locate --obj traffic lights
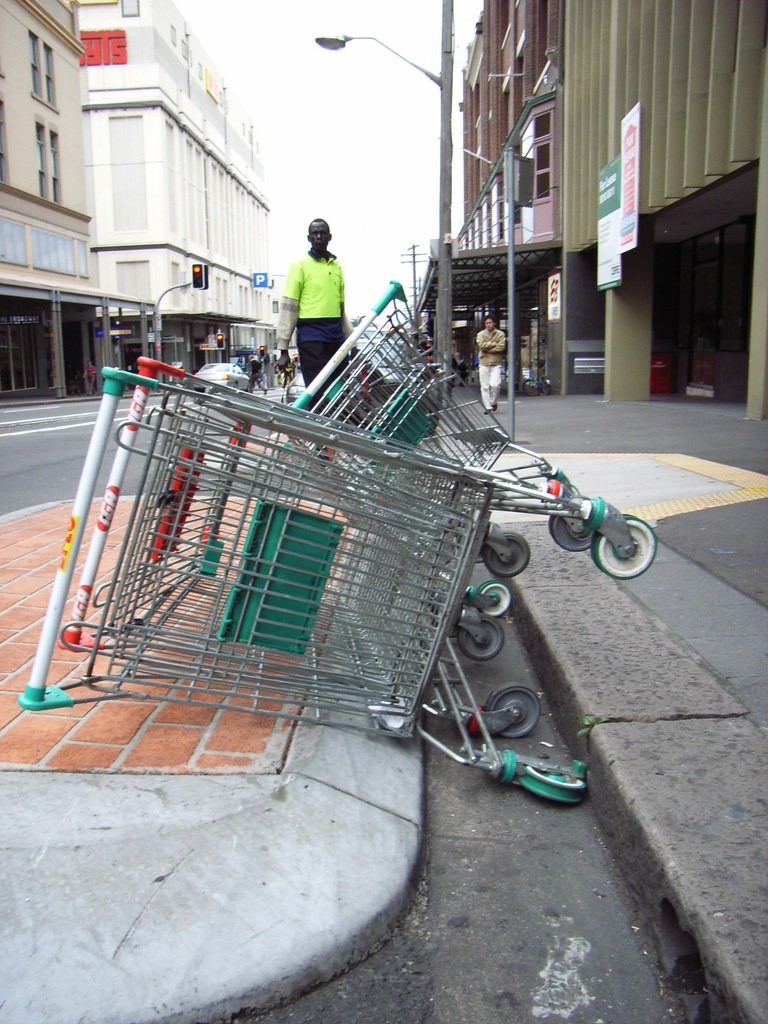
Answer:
[192,263,204,289]
[217,333,224,350]
[259,345,266,357]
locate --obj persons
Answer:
[476,315,505,414]
[276,218,360,468]
[4,363,9,389]
[248,354,267,395]
[68,375,81,395]
[82,361,96,395]
[451,353,467,388]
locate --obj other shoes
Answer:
[492,405,497,411]
[484,410,491,414]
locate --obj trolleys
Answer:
[15,283,665,810]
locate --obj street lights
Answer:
[314,34,454,396]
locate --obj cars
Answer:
[193,362,251,393]
